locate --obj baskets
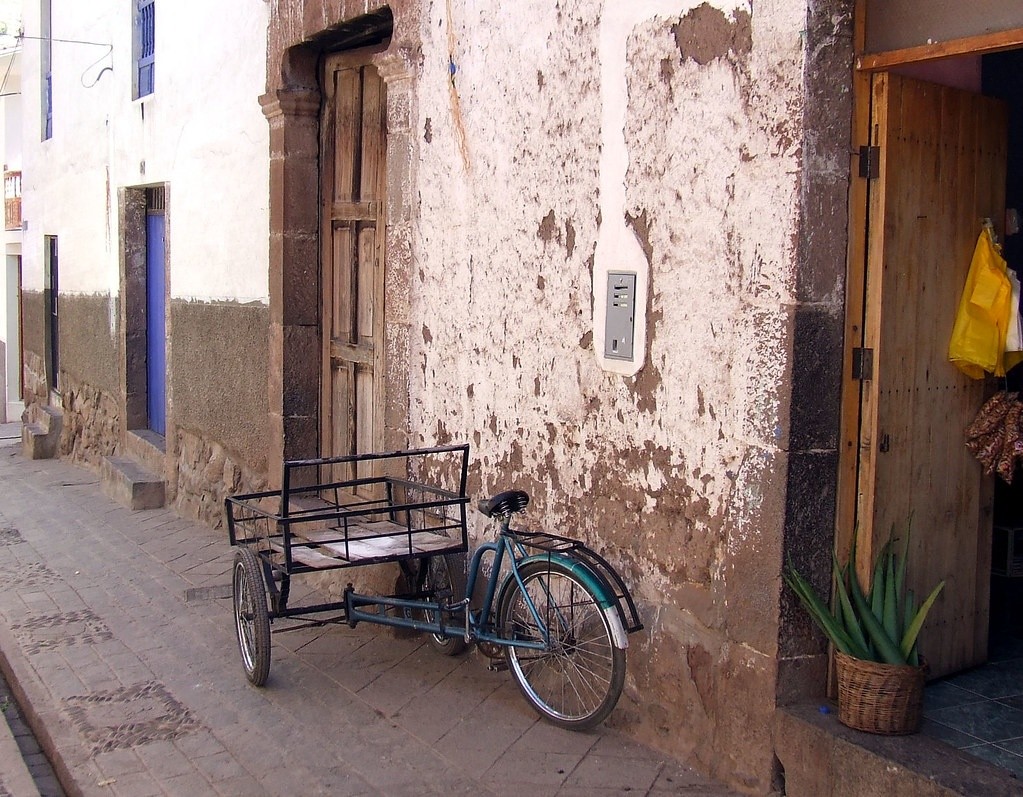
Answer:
[832,646,930,737]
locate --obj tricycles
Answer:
[222,443,644,733]
